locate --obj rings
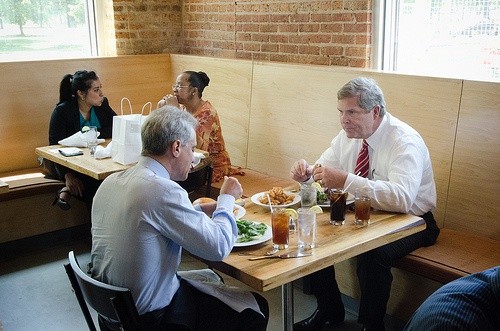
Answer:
[320,179,324,185]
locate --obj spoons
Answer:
[238,248,278,256]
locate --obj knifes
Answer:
[249,254,310,260]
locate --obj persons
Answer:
[290,77,440,331]
[40,71,120,213]
[86,104,269,331]
[157,70,231,184]
[404,267,500,331]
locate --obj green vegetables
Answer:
[316,191,328,205]
[235,219,266,243]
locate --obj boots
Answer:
[360,323,385,331]
[293,291,344,331]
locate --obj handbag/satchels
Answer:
[112,96,151,167]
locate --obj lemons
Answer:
[309,206,323,213]
[311,182,321,191]
[82,126,90,131]
[285,209,298,219]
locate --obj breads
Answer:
[193,198,214,205]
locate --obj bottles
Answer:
[86,126,97,156]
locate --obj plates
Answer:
[233,221,272,248]
[233,203,247,220]
[250,191,301,210]
[317,189,356,207]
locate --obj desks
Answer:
[35,138,209,179]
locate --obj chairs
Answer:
[63,250,142,331]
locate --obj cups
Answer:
[300,187,317,208]
[329,189,348,226]
[297,207,316,250]
[353,188,373,225]
[271,207,290,250]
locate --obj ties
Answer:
[354,139,368,178]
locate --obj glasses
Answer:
[172,84,193,90]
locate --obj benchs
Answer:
[0,54,500,320]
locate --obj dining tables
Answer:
[187,185,426,331]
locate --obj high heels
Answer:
[51,187,72,210]
